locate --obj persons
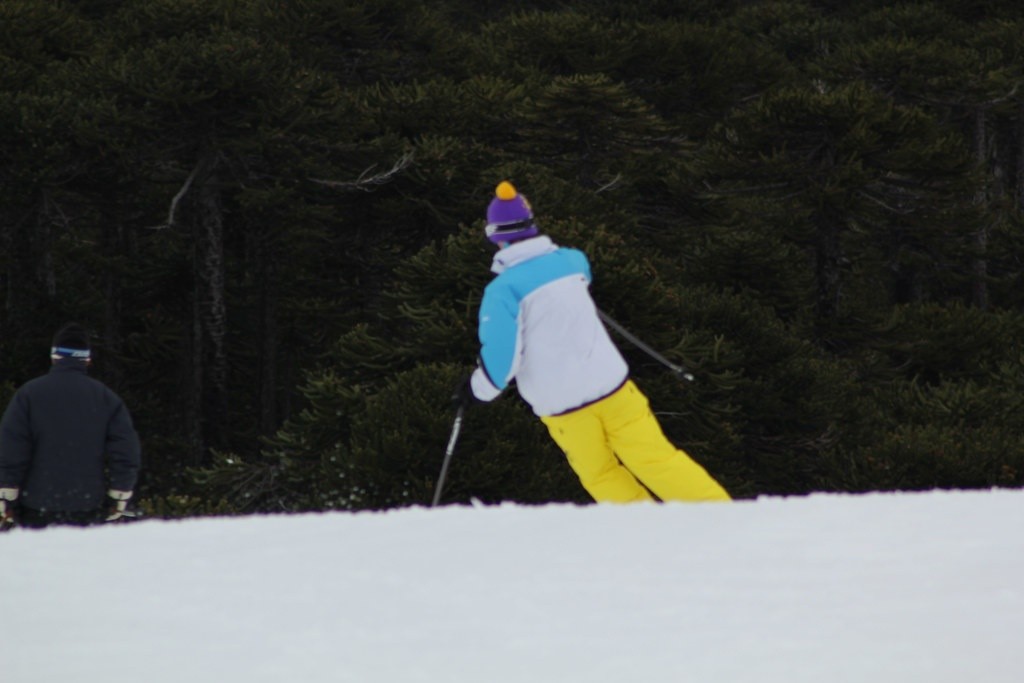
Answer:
[1,325,142,530]
[452,182,733,503]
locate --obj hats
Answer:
[484,180,537,243]
[49,324,91,361]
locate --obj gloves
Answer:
[0,499,14,523]
[103,500,128,521]
